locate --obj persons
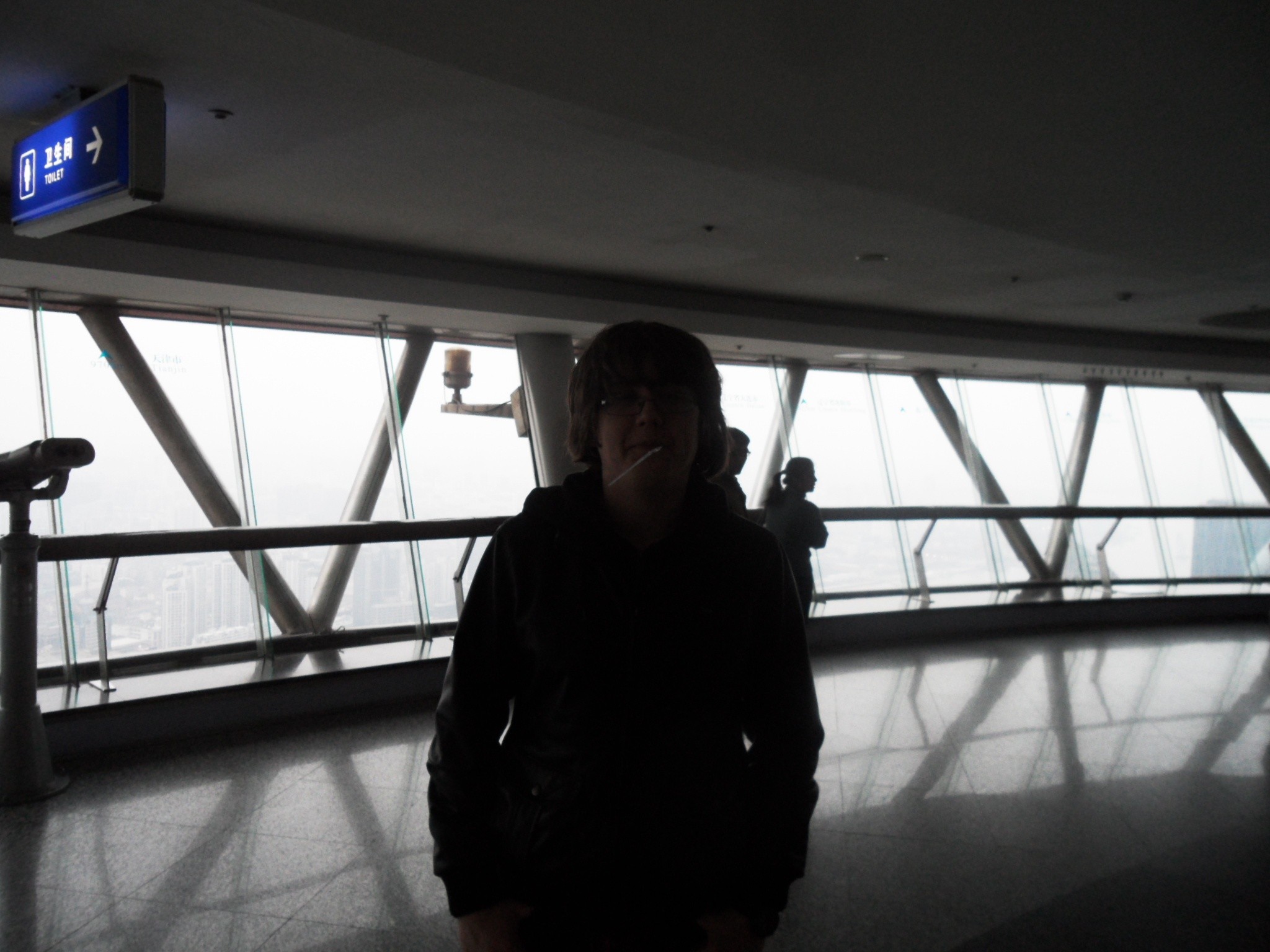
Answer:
[423,319,828,952]
[721,424,752,522]
[761,456,828,628]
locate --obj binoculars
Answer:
[0,437,97,807]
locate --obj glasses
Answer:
[597,390,701,417]
[731,448,751,458]
